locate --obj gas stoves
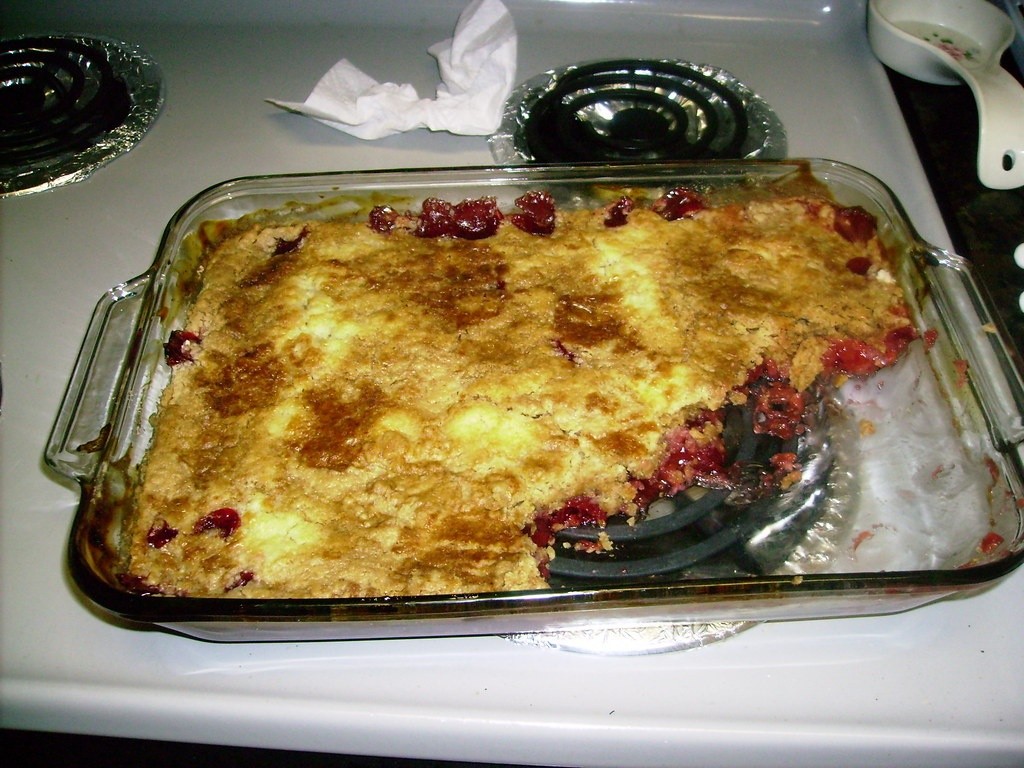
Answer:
[1,1,1022,767]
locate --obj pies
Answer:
[124,187,918,597]
